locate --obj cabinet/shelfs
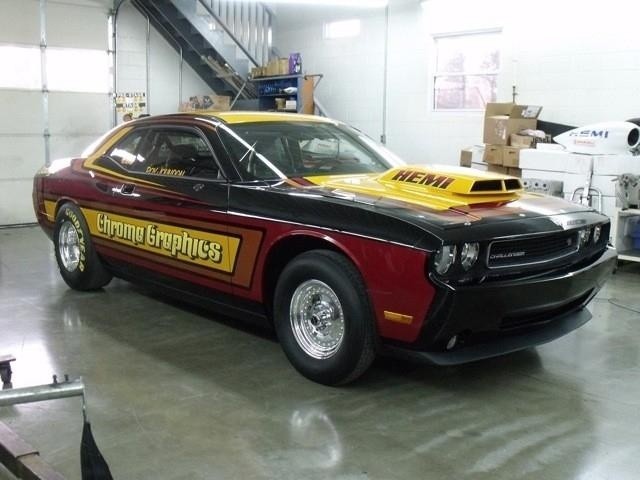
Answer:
[250,75,315,115]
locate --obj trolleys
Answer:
[568,184,639,276]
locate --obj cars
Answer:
[28,107,623,391]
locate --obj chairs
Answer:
[167,143,198,170]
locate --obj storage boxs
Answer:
[481,103,543,178]
[266,54,290,76]
[180,94,230,112]
